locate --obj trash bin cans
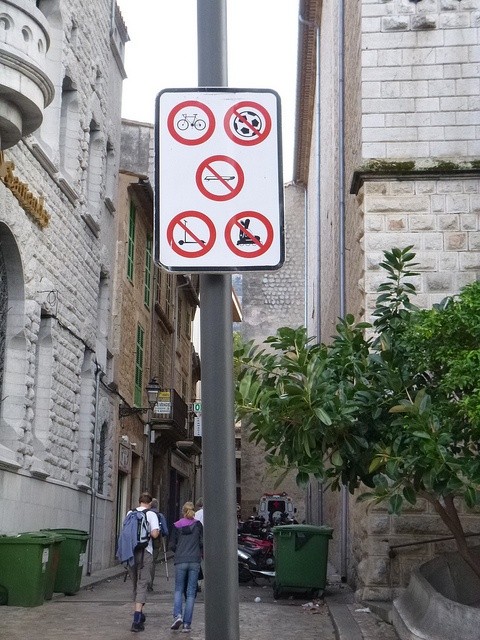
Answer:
[0,531,66,607]
[40,528,89,593]
[271,525,334,600]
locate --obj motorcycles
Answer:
[234,515,309,585]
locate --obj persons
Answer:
[195,498,203,526]
[114,493,161,632]
[146,498,168,592]
[169,501,203,632]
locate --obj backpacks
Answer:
[152,512,163,549]
[131,508,151,550]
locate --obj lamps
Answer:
[118,374,160,417]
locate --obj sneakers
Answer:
[170,614,183,629]
[181,623,192,632]
[130,622,144,632]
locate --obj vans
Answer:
[256,491,297,523]
